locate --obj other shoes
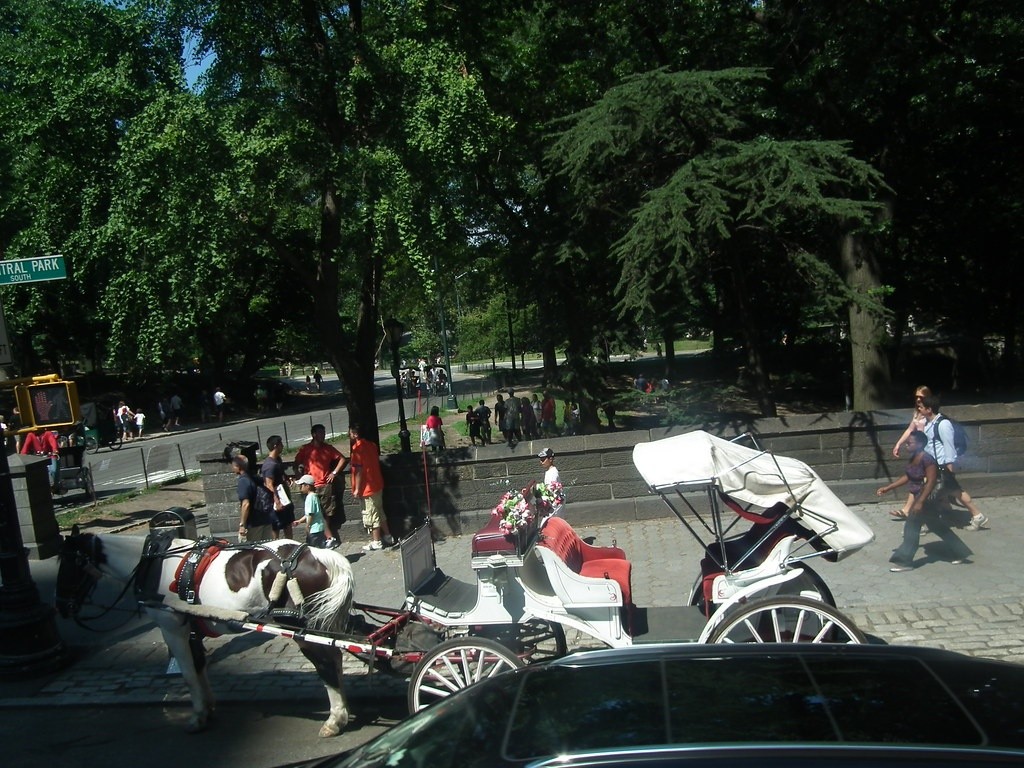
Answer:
[175,424,180,426]
[890,564,914,572]
[951,558,965,564]
[164,428,169,431]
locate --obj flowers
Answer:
[491,480,564,535]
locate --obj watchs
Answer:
[333,471,336,476]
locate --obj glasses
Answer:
[907,441,917,445]
[914,396,923,399]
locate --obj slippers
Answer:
[889,510,908,518]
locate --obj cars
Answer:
[273,638,1024,768]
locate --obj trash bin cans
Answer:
[149,507,197,541]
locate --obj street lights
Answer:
[454,268,479,371]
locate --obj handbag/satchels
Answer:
[921,454,960,502]
[250,486,274,513]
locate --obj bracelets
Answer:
[239,523,243,527]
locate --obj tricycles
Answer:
[25,443,95,500]
[398,365,421,399]
[65,401,124,456]
[422,363,450,397]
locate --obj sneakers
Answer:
[918,525,929,536]
[963,513,988,531]
[325,537,339,549]
[363,541,382,550]
[382,534,394,545]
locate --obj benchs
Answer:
[540,519,632,636]
[700,501,793,621]
[472,479,536,565]
[58,446,87,477]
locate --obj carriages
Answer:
[53,430,876,739]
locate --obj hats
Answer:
[508,388,514,393]
[295,475,314,485]
[537,447,555,457]
[123,408,127,414]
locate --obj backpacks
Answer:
[934,415,967,456]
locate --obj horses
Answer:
[52,525,355,739]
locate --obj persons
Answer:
[537,448,561,484]
[400,371,417,388]
[9,407,23,454]
[643,338,648,352]
[162,392,183,431]
[426,370,447,386]
[306,370,323,392]
[254,383,284,416]
[425,405,444,450]
[295,424,347,550]
[877,385,989,572]
[349,422,396,550]
[20,429,59,478]
[199,387,227,423]
[636,373,669,395]
[232,455,273,543]
[466,387,579,446]
[293,475,326,546]
[261,436,296,539]
[113,401,145,440]
